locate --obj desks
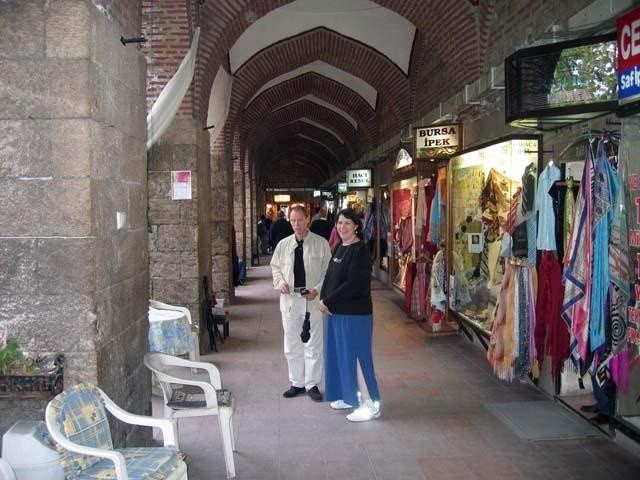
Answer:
[148,306,199,387]
[214,311,230,340]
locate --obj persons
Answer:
[256,205,342,257]
[318,207,382,422]
[270,205,333,403]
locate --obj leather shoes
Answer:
[581,404,598,411]
[590,413,612,424]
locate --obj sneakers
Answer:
[330,392,362,409]
[346,398,381,422]
[283,386,306,397]
[307,386,322,402]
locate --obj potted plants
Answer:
[0,335,65,398]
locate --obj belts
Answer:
[294,287,306,293]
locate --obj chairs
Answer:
[45,384,193,480]
[149,296,192,331]
[143,352,242,478]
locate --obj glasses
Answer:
[332,246,349,264]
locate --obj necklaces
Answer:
[333,236,357,263]
